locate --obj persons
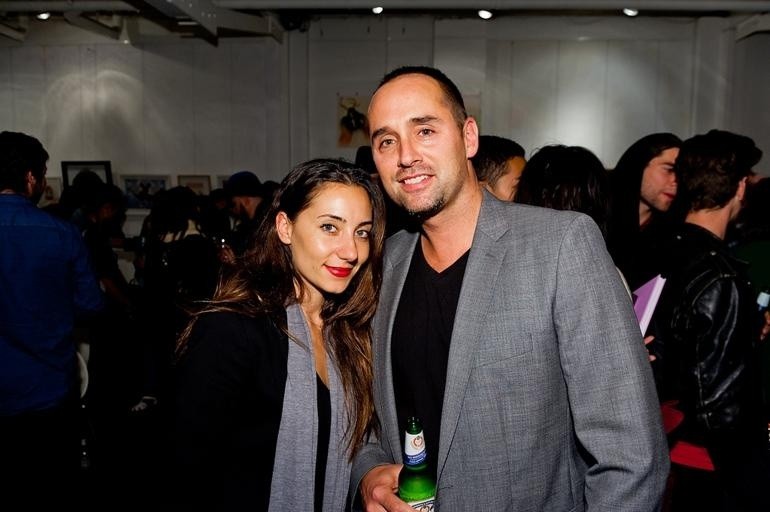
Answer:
[141,184,218,314]
[0,129,101,416]
[160,159,655,512]
[657,129,770,435]
[217,169,267,266]
[39,168,104,226]
[73,183,159,413]
[478,134,526,203]
[351,65,671,512]
[513,143,607,218]
[612,130,680,227]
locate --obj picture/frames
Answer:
[119,173,172,209]
[177,175,213,198]
[59,160,113,193]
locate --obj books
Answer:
[632,273,666,335]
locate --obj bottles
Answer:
[398,417,436,512]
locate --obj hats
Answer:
[224,172,261,197]
